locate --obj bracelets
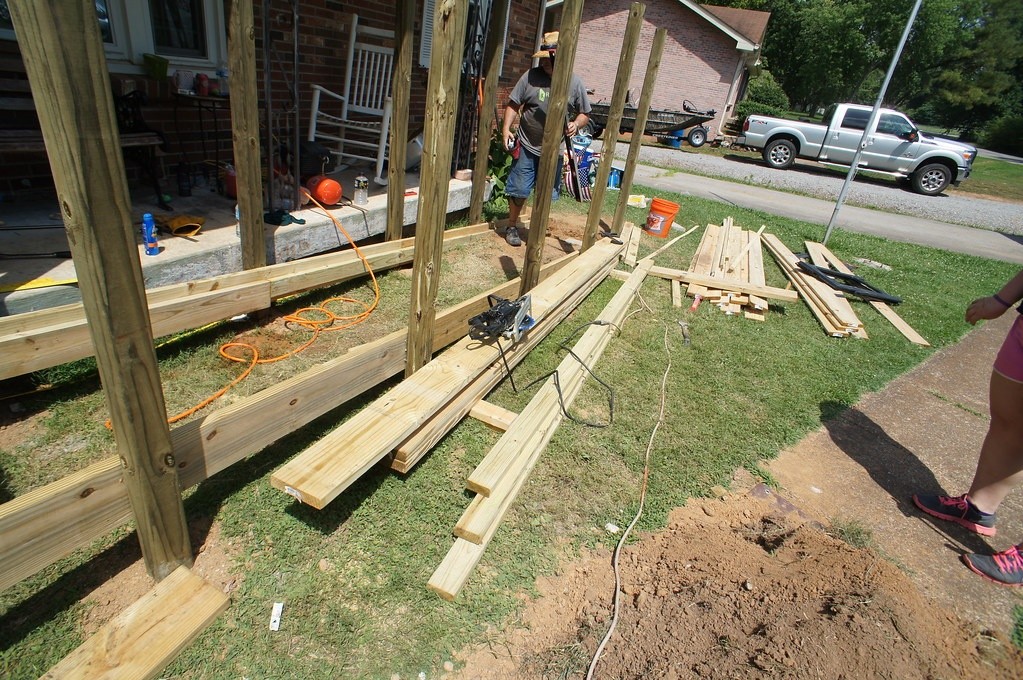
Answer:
[993,294,1012,309]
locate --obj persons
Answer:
[912,266,1023,587]
[499,30,592,247]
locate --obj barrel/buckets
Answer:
[644,198,681,239]
[665,128,684,148]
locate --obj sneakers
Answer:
[962,544,1023,585]
[914,492,996,537]
[505,226,521,245]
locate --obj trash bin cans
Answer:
[663,130,684,148]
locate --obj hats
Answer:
[532,31,560,57]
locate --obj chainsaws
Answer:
[467,294,535,343]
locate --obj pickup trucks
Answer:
[733,102,979,196]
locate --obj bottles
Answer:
[177,158,192,197]
[216,58,230,96]
[280,181,295,213]
[354,172,369,206]
[235,203,241,238]
[141,214,159,255]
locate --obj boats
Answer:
[595,88,716,135]
